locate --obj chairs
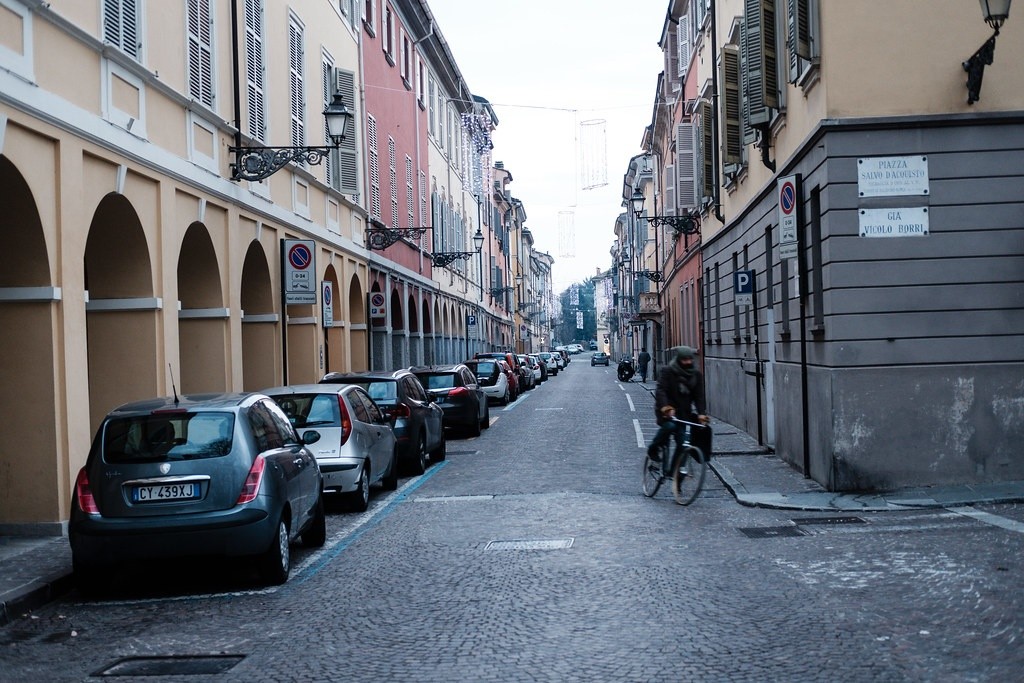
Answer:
[138,421,175,451]
[219,419,228,437]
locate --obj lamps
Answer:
[518,290,542,311]
[489,272,522,297]
[629,187,701,235]
[530,303,564,329]
[431,229,485,268]
[229,89,354,182]
[612,284,634,303]
[622,254,664,282]
[962,0,1012,105]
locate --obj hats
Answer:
[677,345,693,359]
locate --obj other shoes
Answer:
[647,445,661,462]
[679,466,688,476]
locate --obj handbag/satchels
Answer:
[688,420,713,463]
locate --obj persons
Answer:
[646,345,709,492]
[638,348,650,383]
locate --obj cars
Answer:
[259,383,398,512]
[317,368,446,476]
[409,365,490,437]
[462,344,585,406]
[590,352,609,366]
[68,391,325,584]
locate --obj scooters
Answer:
[617,358,633,382]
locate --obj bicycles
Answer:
[642,415,710,506]
